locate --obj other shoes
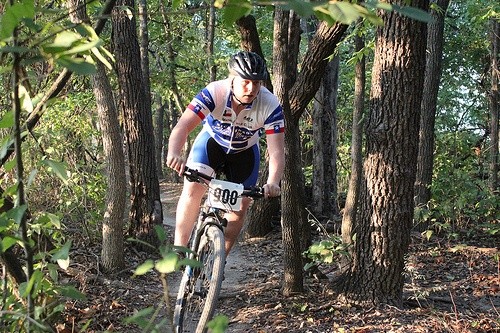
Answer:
[207,255,226,280]
[171,249,187,271]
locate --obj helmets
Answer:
[229,50,269,80]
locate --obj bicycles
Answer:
[169,163,276,333]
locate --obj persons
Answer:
[164,51,286,280]
